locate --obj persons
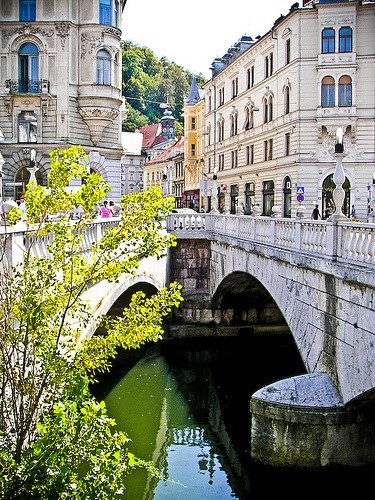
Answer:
[200,206,206,213]
[18,198,28,222]
[310,204,322,220]
[71,204,84,220]
[350,205,356,219]
[324,206,333,218]
[92,201,120,219]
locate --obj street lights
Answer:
[26,148,40,185]
[210,166,219,213]
[328,127,350,222]
